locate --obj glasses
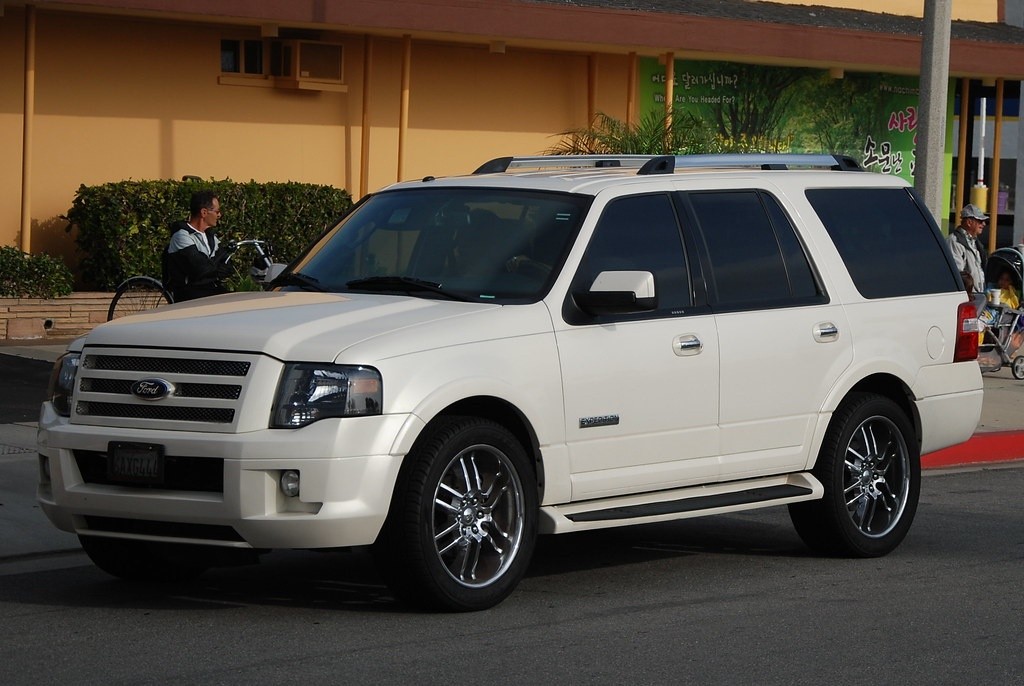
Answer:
[205,208,220,214]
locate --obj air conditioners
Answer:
[280,38,344,84]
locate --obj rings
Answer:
[514,255,516,260]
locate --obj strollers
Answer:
[972,246,1024,380]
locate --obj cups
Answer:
[989,288,1001,305]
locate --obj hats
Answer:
[960,203,989,220]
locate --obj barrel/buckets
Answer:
[969,187,988,214]
[998,185,1009,212]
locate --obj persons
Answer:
[978,273,1020,356]
[945,205,989,293]
[433,208,633,307]
[162,191,233,305]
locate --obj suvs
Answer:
[34,150,984,615]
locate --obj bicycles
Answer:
[107,239,276,321]
